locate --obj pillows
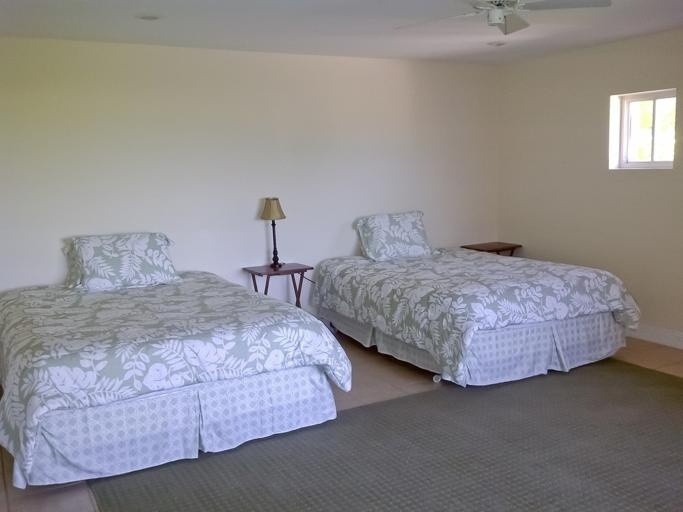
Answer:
[62,233,184,293]
[356,210,434,262]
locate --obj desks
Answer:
[464,242,522,257]
[243,263,314,309]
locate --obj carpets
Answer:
[85,357,683,510]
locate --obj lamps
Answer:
[261,197,287,268]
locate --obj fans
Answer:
[394,0,612,37]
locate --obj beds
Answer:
[0,270,352,490]
[311,247,641,388]
